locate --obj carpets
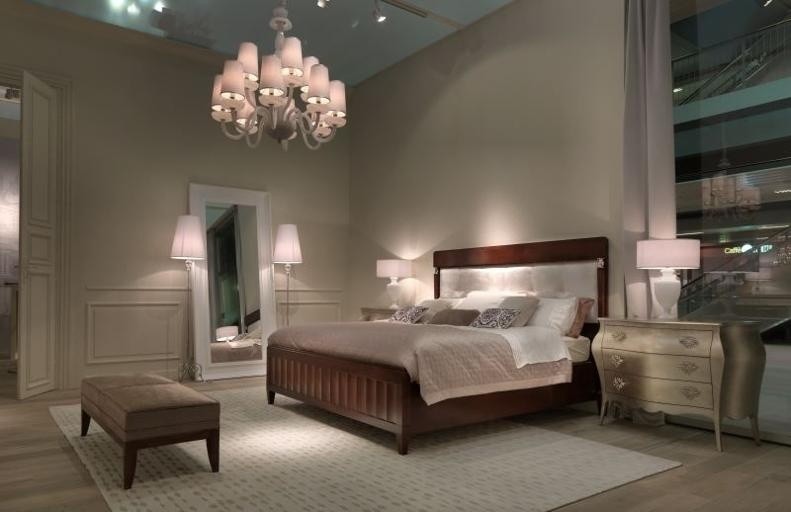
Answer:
[50,383,683,512]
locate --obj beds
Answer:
[210,309,262,362]
[266,237,610,457]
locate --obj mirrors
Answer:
[188,183,277,380]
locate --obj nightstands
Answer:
[592,318,766,452]
[360,307,400,322]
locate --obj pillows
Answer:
[525,297,578,334]
[232,333,244,342]
[243,328,262,340]
[387,305,427,324]
[470,308,522,328]
[418,300,451,324]
[426,309,481,325]
[438,297,463,308]
[499,296,539,327]
[566,297,595,338]
[459,289,521,311]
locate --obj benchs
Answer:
[79,374,221,490]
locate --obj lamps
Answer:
[317,1,334,10]
[637,240,701,319]
[702,241,759,318]
[272,224,303,327]
[371,0,387,23]
[210,0,347,152]
[216,327,237,342]
[169,215,208,382]
[376,260,410,310]
